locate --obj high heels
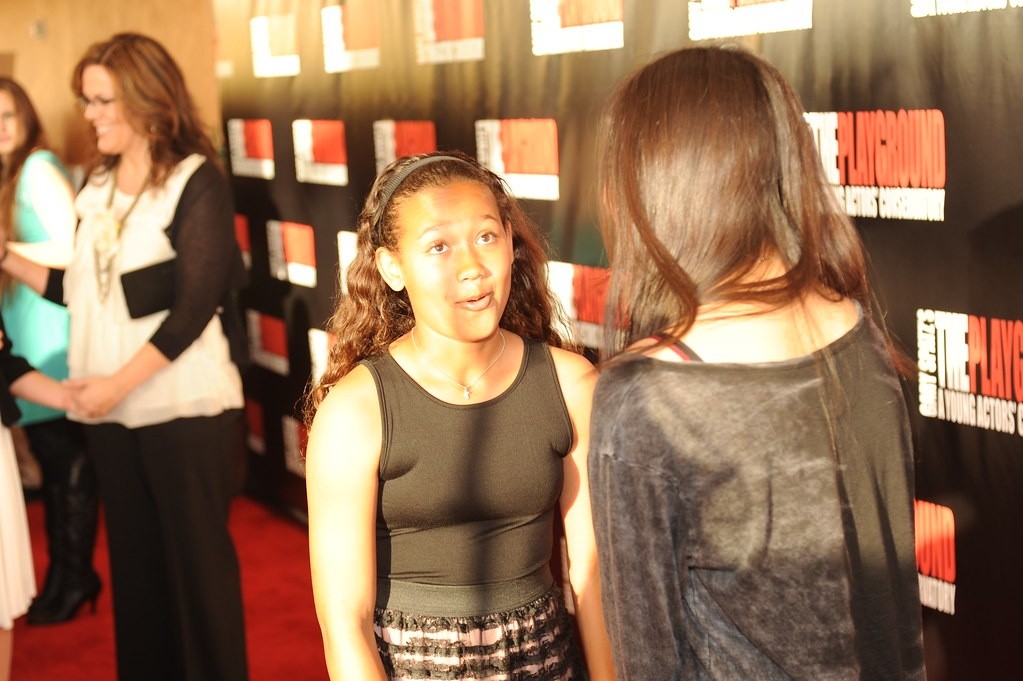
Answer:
[27,567,101,626]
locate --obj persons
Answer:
[0,33,250,681]
[0,78,103,626]
[306,150,617,680]
[588,47,927,681]
[0,318,88,681]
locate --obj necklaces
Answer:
[411,325,506,400]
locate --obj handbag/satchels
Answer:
[120,243,248,320]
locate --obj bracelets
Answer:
[0,249,8,263]
